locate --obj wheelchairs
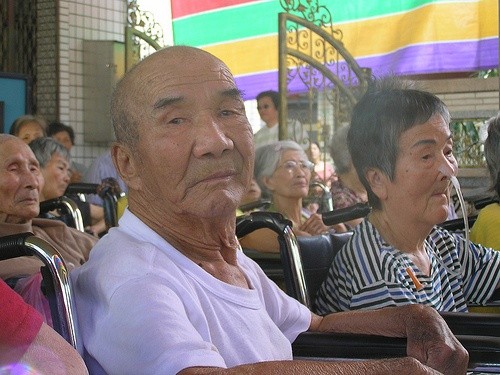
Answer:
[234,201,500,333]
[1,233,500,374]
[39,182,118,233]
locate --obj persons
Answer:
[69,46,468,375]
[230,89,373,254]
[0,113,128,375]
[312,73,499,314]
[465,114,500,314]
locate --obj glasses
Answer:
[276,159,315,173]
[257,103,275,109]
